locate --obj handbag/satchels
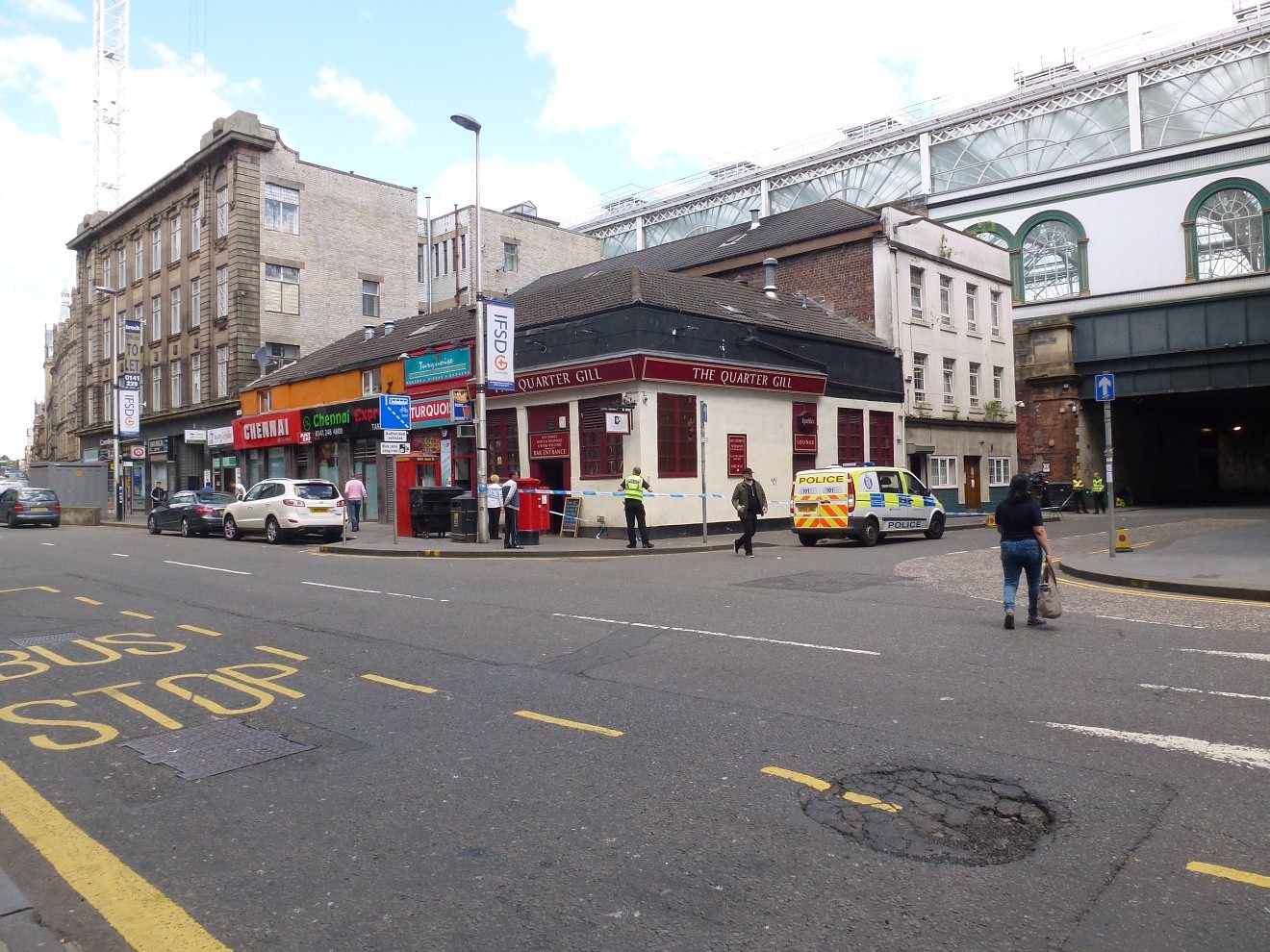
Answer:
[1036,558,1063,620]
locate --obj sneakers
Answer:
[1028,615,1046,625]
[744,550,755,558]
[356,527,359,531]
[492,536,502,540]
[734,538,742,555]
[1004,613,1015,629]
[490,536,496,539]
[351,526,357,532]
[626,543,637,548]
[642,541,655,548]
[503,542,513,549]
[512,542,525,549]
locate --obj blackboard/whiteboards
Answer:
[561,497,582,531]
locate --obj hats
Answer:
[741,468,754,475]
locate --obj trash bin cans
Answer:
[1043,481,1076,512]
[450,496,478,544]
[1028,483,1045,508]
[408,486,463,538]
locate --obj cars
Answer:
[146,490,237,537]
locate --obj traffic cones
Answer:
[1114,527,1133,552]
[986,511,997,529]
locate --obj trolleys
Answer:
[409,486,465,539]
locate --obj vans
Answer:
[788,461,946,547]
[0,486,61,528]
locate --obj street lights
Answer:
[96,286,121,519]
[450,112,493,544]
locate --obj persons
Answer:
[995,474,1052,630]
[617,466,655,548]
[1090,472,1106,514]
[151,481,166,509]
[1072,474,1089,514]
[487,473,505,540]
[343,474,368,533]
[201,482,213,491]
[228,482,247,501]
[731,468,768,558]
[500,471,524,549]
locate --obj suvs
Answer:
[222,476,349,544]
